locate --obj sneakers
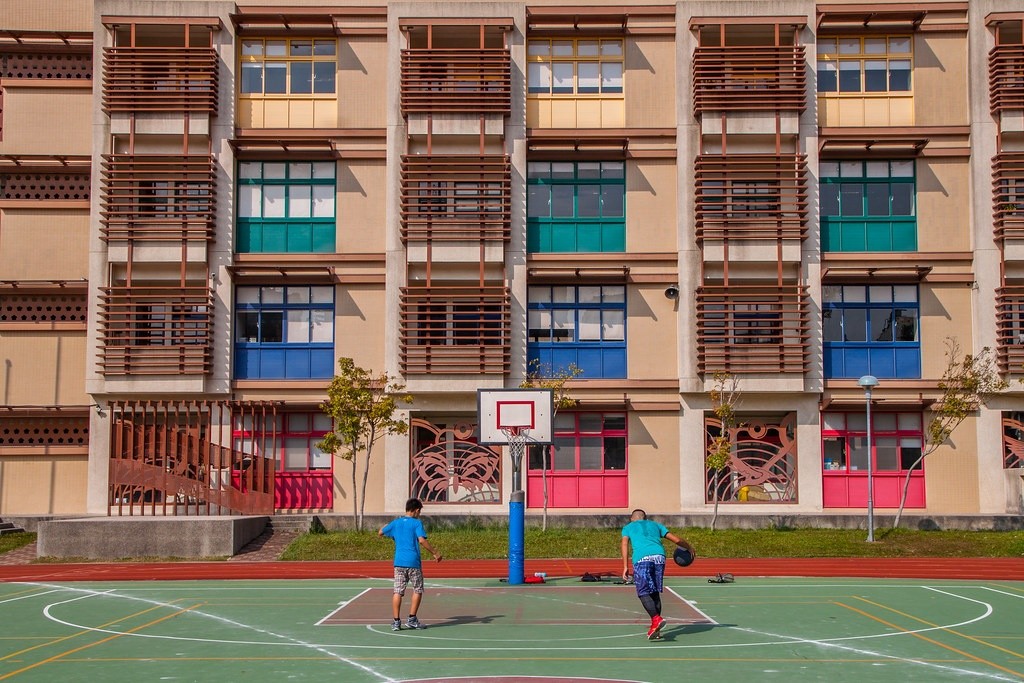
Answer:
[655,633,661,639]
[390,619,404,631]
[647,615,667,640]
[405,615,429,630]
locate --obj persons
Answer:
[378,499,442,631]
[621,509,696,640]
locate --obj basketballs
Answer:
[673,545,695,567]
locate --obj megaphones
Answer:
[665,284,679,299]
